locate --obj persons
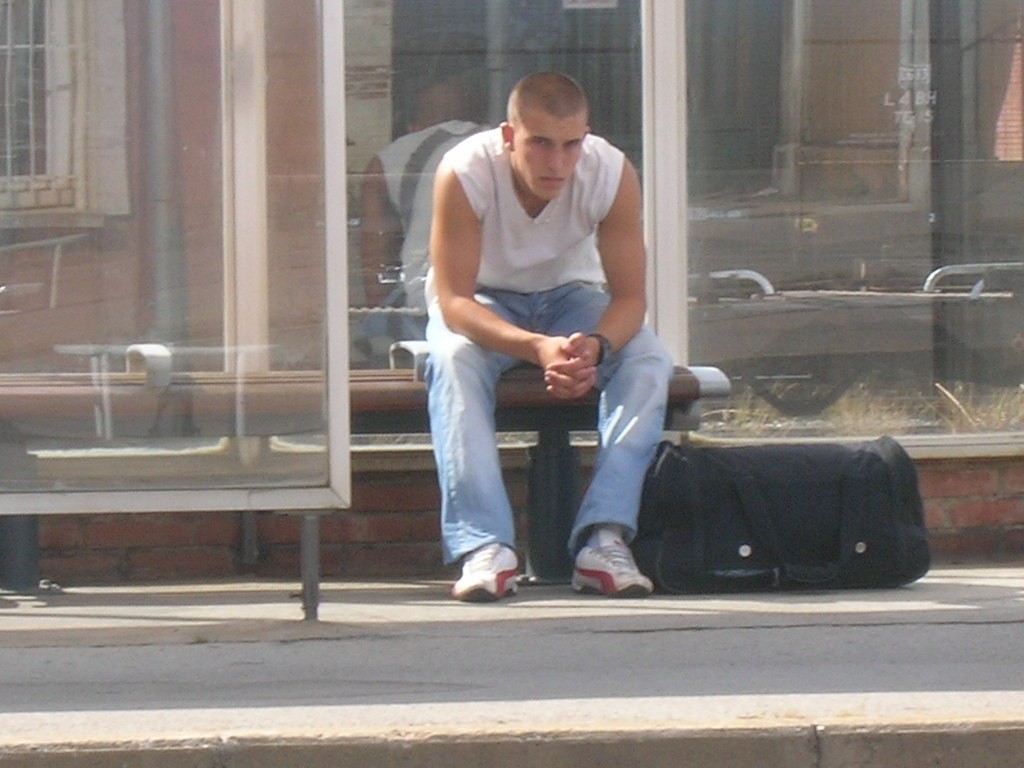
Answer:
[424,72,676,602]
[348,67,498,369]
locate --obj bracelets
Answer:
[588,334,611,366]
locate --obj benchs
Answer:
[0,364,731,583]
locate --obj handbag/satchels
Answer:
[631,437,931,590]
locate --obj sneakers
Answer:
[570,526,655,598]
[452,543,520,600]
